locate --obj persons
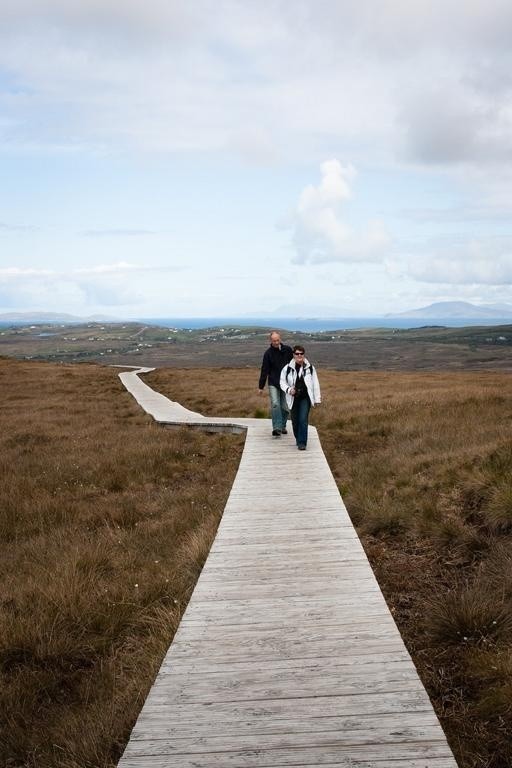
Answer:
[257,332,322,450]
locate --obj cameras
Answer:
[292,384,306,400]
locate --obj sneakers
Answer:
[272,429,287,436]
[298,445,306,450]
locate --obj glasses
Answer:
[295,353,304,355]
